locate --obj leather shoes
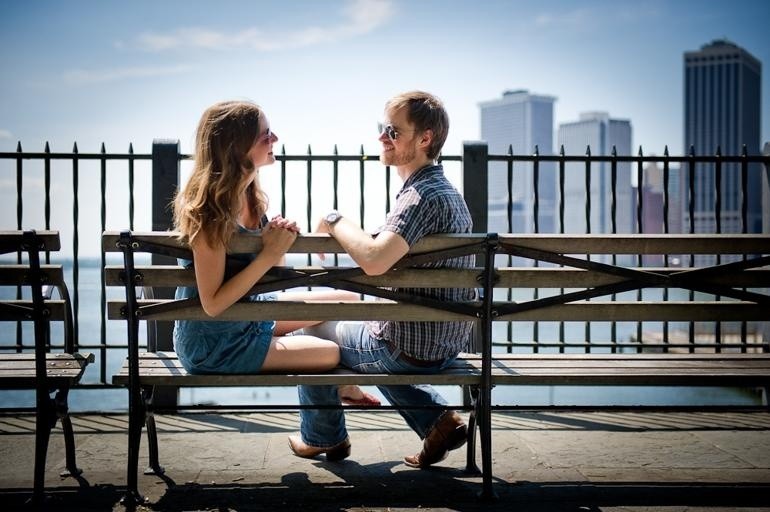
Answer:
[402,410,468,468]
[339,394,381,406]
[288,435,351,462]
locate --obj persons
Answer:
[173,102,380,407]
[289,92,476,467]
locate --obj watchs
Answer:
[323,212,343,238]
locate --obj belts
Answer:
[384,340,454,367]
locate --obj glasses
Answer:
[376,121,419,142]
[257,128,272,145]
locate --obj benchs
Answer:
[1,230,96,490]
[100,230,769,504]
